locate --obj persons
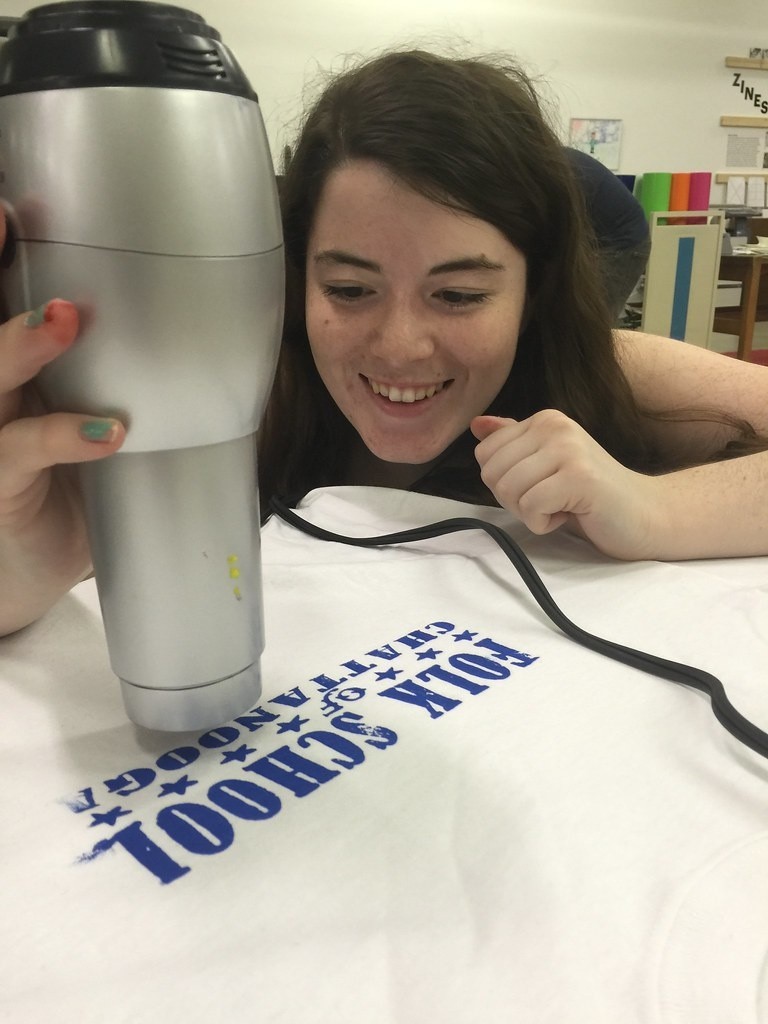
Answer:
[0,50,768,635]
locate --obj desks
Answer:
[712,250,768,364]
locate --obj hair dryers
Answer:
[0,2,285,740]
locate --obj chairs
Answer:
[731,217,768,238]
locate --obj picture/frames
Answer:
[569,116,624,172]
